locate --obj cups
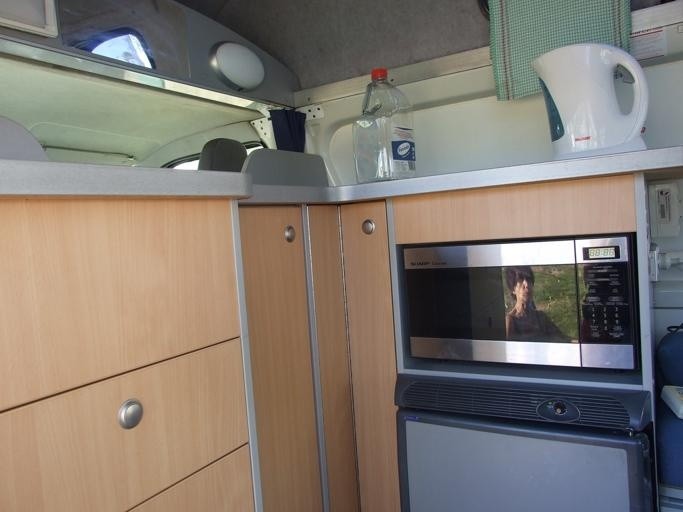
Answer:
[529,42,650,163]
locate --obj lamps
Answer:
[207,38,265,94]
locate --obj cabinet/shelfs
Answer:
[232,204,327,511]
[0,195,269,511]
[332,199,406,512]
[305,204,360,510]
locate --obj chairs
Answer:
[652,325,683,510]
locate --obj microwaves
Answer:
[398,233,639,373]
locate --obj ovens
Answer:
[389,372,659,511]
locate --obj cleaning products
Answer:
[662,383,682,416]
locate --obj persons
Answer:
[504,267,576,342]
